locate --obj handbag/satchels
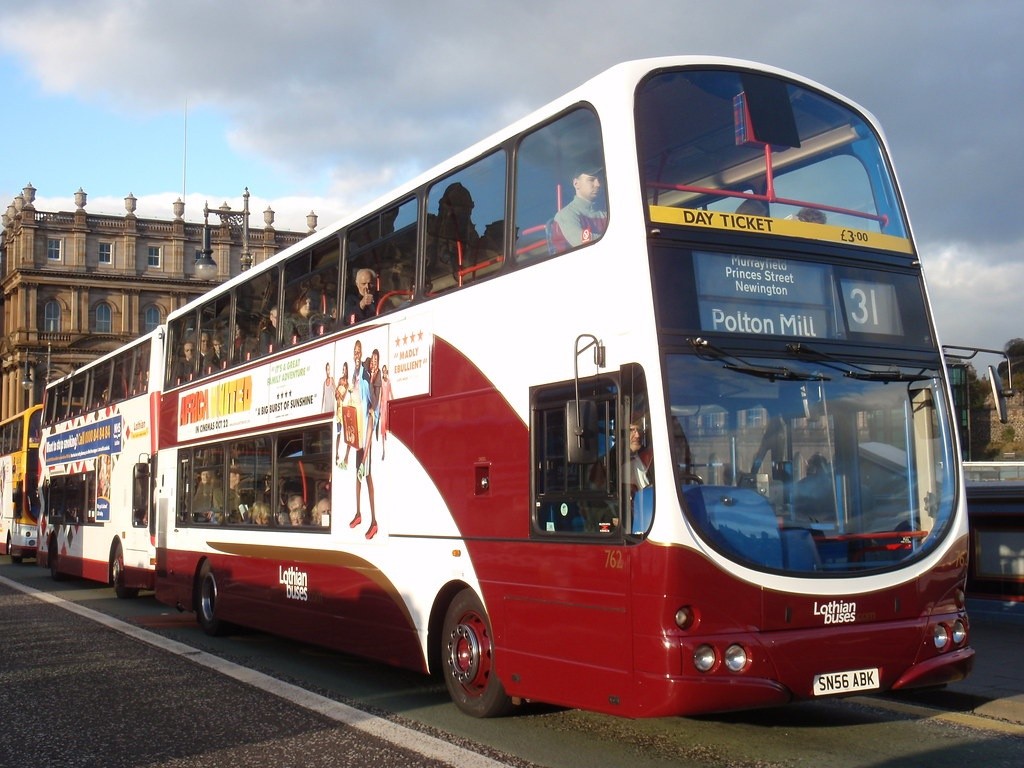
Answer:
[342,390,359,450]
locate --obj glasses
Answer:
[630,427,646,435]
[185,348,194,352]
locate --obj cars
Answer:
[959,477,1023,624]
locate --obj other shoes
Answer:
[338,460,348,469]
[382,451,385,460]
[358,463,366,482]
[350,514,361,528]
[336,456,340,465]
[365,521,377,539]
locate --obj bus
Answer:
[155,53,1015,719]
[36,324,165,599]
[0,401,89,564]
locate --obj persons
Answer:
[321,340,394,540]
[172,159,610,384]
[189,468,332,528]
[590,412,652,516]
[735,196,829,224]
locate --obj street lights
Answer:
[194,186,252,281]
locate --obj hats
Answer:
[229,465,243,475]
[568,160,604,184]
